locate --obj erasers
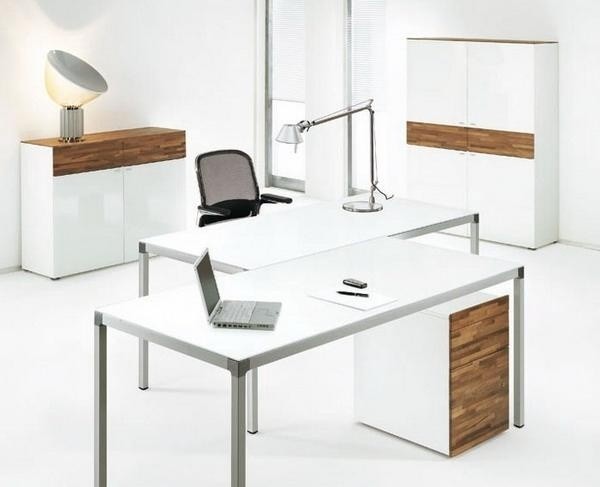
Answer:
[343,279,367,288]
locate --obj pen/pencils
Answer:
[336,291,369,297]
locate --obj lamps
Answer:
[44,50,108,142]
[275,97,383,212]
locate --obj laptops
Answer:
[193,247,282,330]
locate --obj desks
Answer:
[135,195,481,435]
[92,234,525,487]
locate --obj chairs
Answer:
[196,149,292,225]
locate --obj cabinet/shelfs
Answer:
[19,125,186,280]
[404,37,558,250]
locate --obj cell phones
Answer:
[343,277,367,289]
